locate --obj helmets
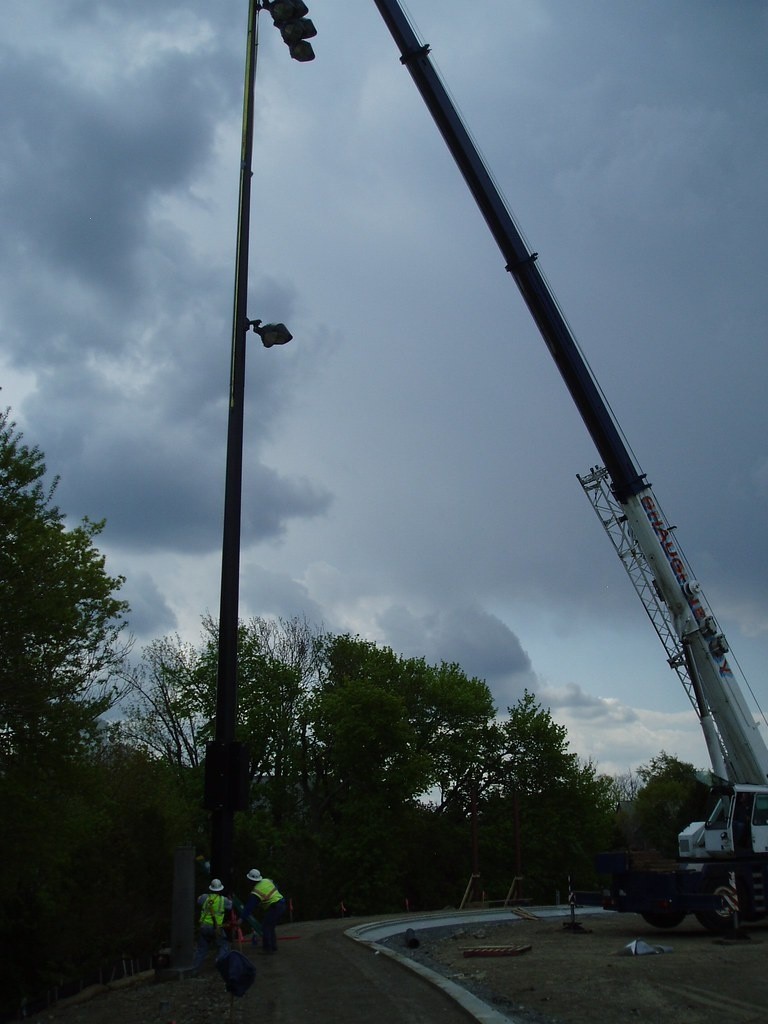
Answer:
[209,879,224,891]
[247,869,262,881]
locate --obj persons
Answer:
[192,879,232,974]
[237,869,286,954]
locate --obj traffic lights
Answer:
[269,0,317,62]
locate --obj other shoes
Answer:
[257,946,278,955]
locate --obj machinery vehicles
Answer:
[373,1,768,931]
